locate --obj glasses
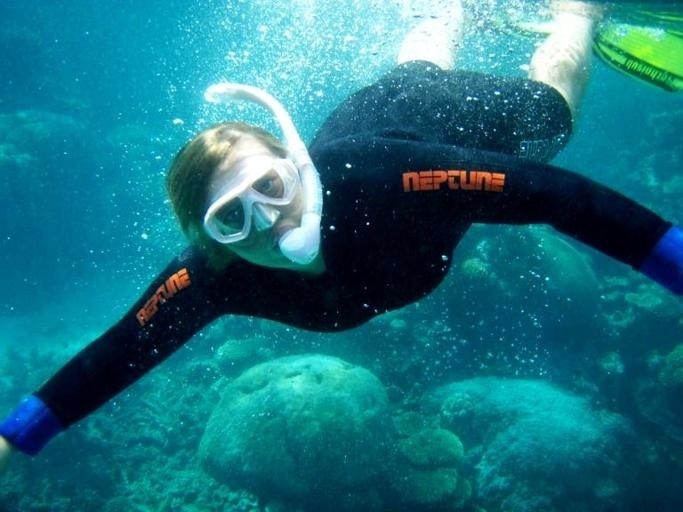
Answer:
[204,159,299,245]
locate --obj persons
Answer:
[2,1,681,482]
